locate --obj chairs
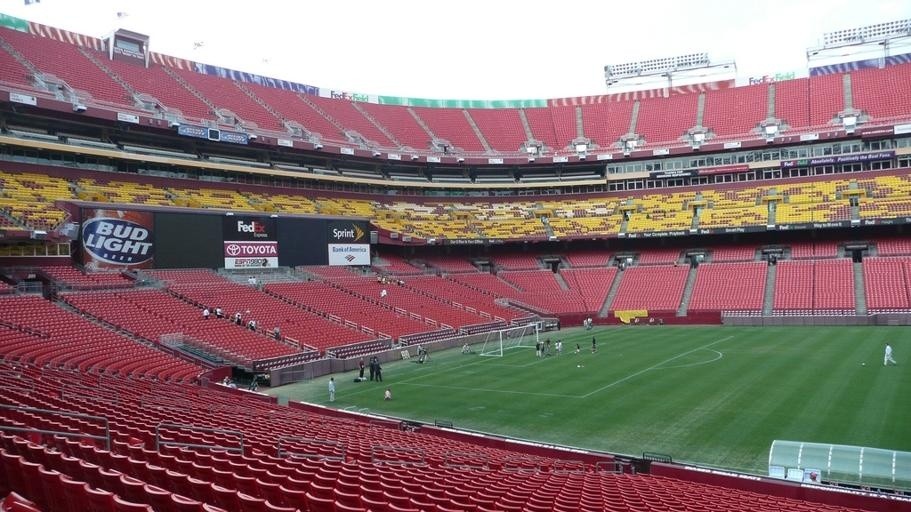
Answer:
[199,393,681,510]
[2,293,213,510]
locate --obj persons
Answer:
[660,318,664,328]
[616,256,633,271]
[574,344,580,354]
[557,322,561,331]
[248,275,263,292]
[592,336,597,354]
[650,316,654,325]
[375,272,405,297]
[328,377,336,402]
[274,325,280,341]
[583,316,593,330]
[635,317,640,326]
[358,355,382,382]
[883,343,897,366]
[416,344,424,363]
[202,306,256,332]
[384,388,392,400]
[222,370,271,392]
[536,337,563,359]
[462,342,472,354]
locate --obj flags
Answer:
[25,0,40,5]
